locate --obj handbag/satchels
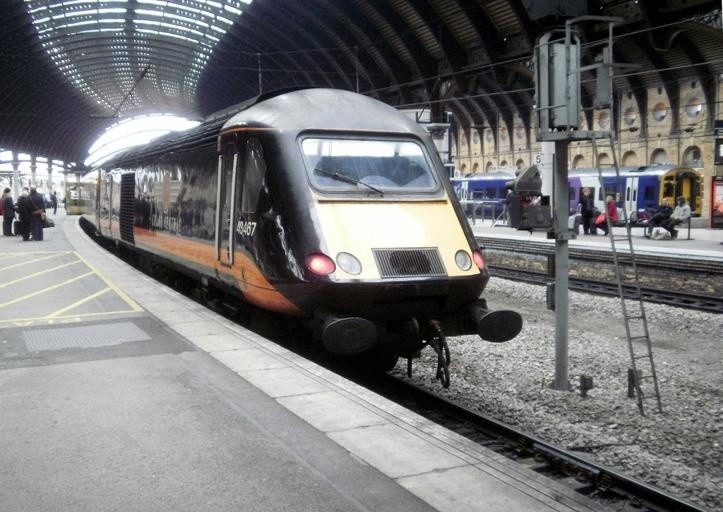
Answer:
[30,209,44,216]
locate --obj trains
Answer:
[78,81,529,386]
[443,164,710,229]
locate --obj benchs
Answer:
[574,212,695,240]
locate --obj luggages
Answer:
[568,204,582,239]
[13,220,24,237]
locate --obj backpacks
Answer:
[0,197,6,216]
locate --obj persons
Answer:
[642,196,673,239]
[135,192,216,239]
[596,194,618,235]
[576,187,598,235]
[660,196,691,240]
[0,185,58,241]
